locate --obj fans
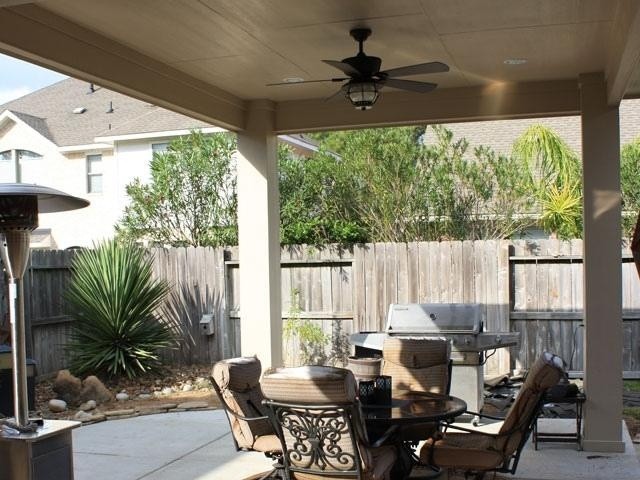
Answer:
[267,27,449,104]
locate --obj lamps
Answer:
[342,78,380,112]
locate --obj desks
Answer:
[354,390,469,479]
[528,390,588,452]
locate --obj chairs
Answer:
[379,335,454,465]
[415,350,566,480]
[258,364,399,480]
[208,354,299,479]
